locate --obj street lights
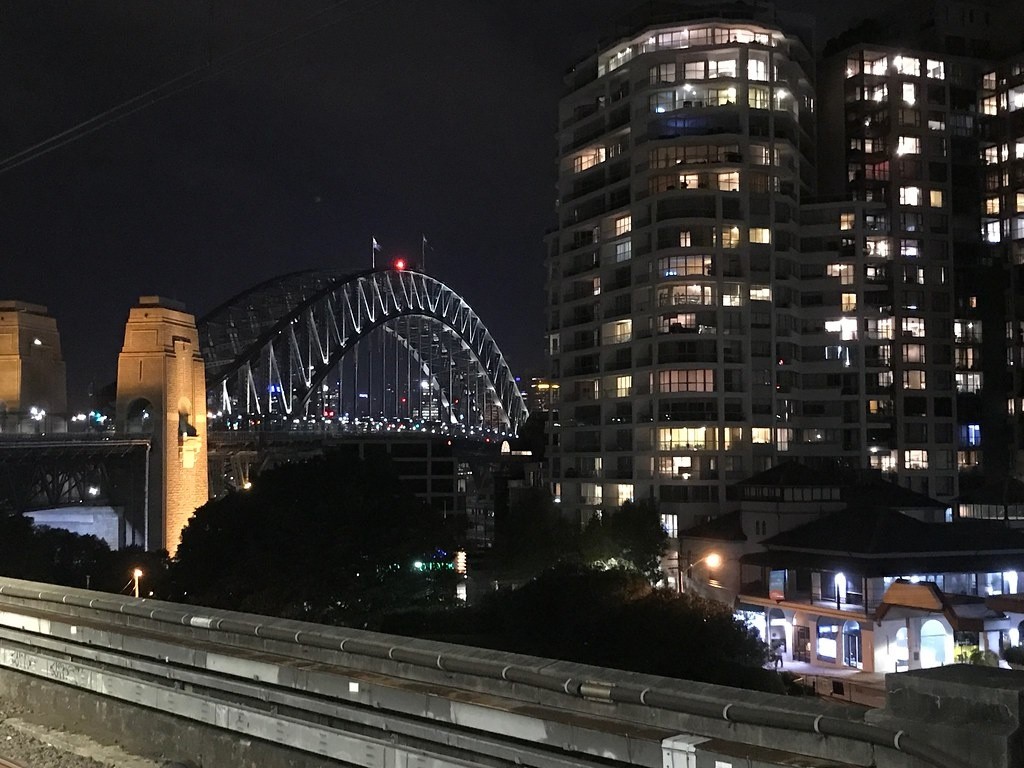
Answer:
[678,553,722,595]
